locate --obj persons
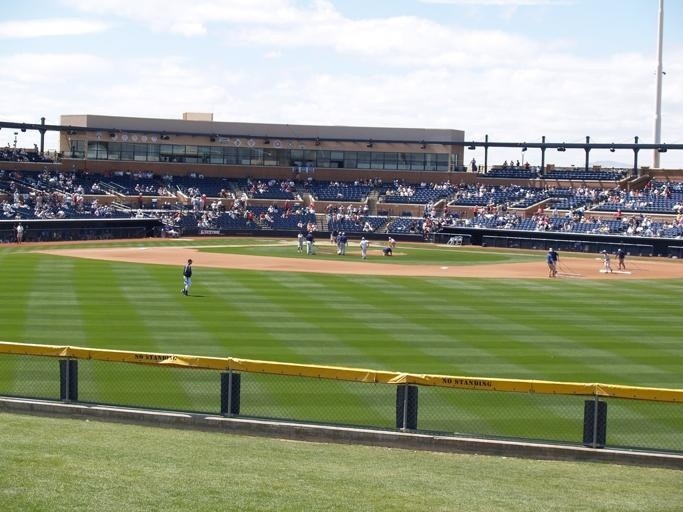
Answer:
[181,258,192,296]
[0,144,682,277]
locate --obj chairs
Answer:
[0,146,682,243]
